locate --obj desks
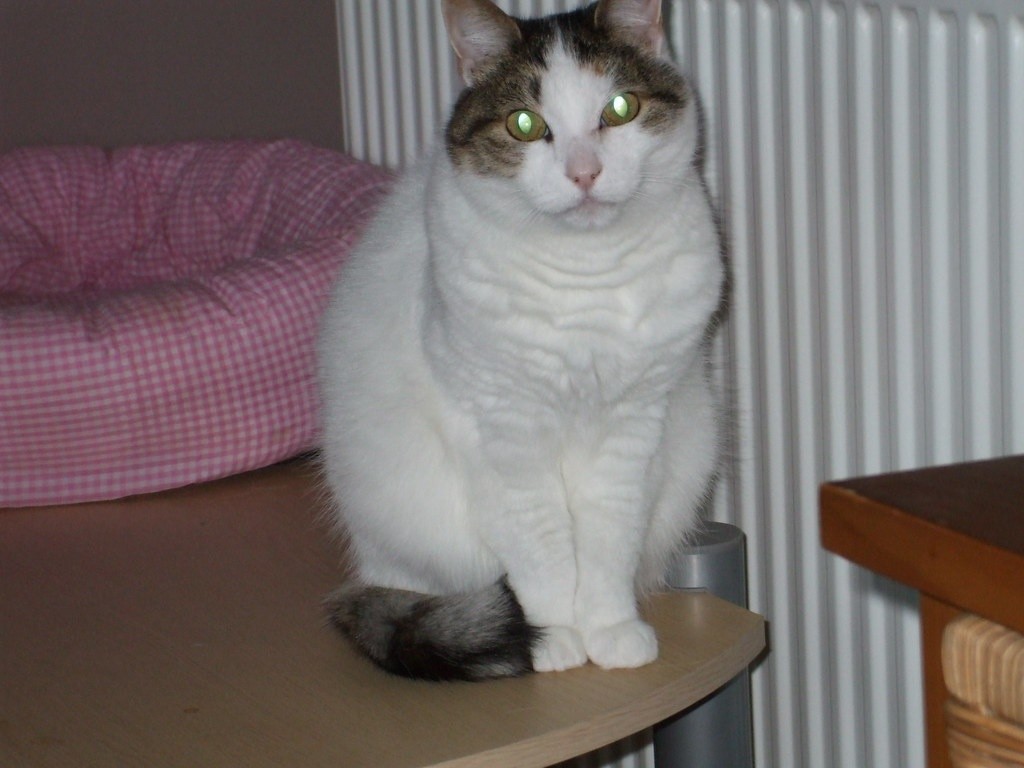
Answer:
[818,454,1023,768]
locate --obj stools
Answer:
[1,453,773,766]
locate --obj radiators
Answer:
[333,0,1023,765]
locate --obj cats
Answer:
[310,0,727,686]
